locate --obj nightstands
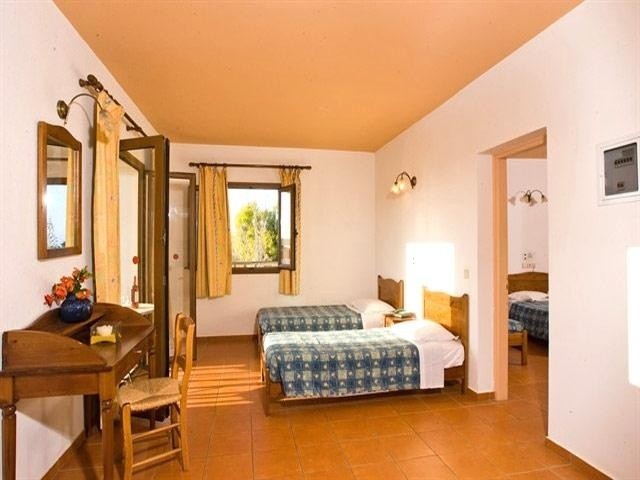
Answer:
[385,316,416,327]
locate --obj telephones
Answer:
[393,309,413,318]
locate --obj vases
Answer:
[59,295,92,323]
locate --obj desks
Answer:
[0,303,157,480]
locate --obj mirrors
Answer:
[37,121,82,259]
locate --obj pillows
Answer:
[391,320,459,343]
[351,298,396,314]
[508,291,549,302]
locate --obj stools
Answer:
[508,330,528,365]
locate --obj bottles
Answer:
[131,276,140,309]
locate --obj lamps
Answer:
[520,190,548,205]
[390,172,416,194]
[58,94,118,131]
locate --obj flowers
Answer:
[44,265,95,308]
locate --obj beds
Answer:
[257,274,403,362]
[508,272,548,341]
[260,287,469,416]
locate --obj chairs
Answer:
[117,312,195,480]
